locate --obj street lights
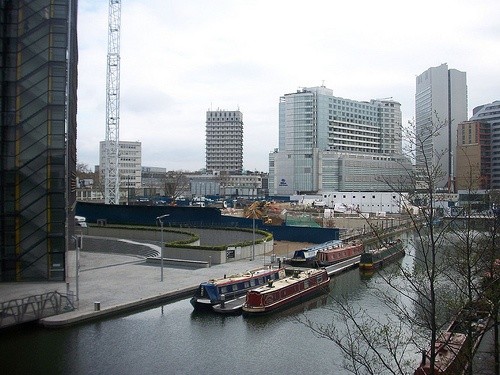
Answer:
[157,214,170,283]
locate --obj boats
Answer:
[190,239,405,314]
[414,293,494,375]
